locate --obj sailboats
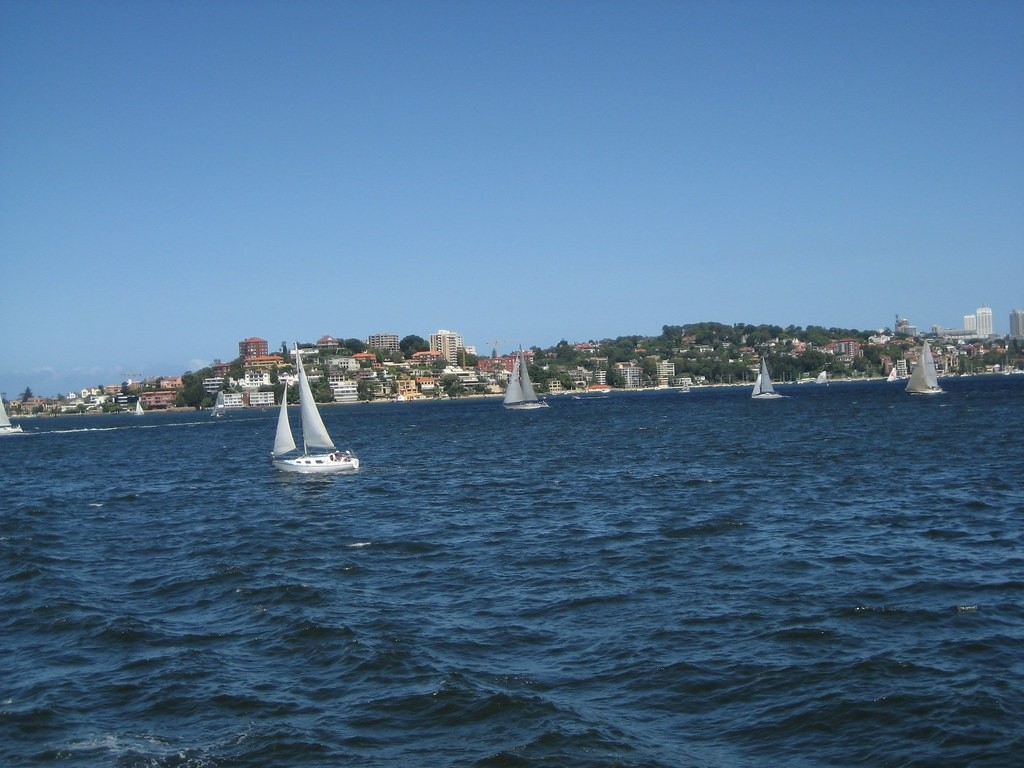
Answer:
[0,393,24,434]
[816,370,828,383]
[887,367,900,381]
[270,340,361,473]
[211,392,225,417]
[750,356,791,398]
[502,344,551,410]
[903,340,945,394]
[134,401,145,415]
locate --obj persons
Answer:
[344,450,351,462]
[334,450,341,462]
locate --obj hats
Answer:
[346,451,350,455]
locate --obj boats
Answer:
[678,384,690,393]
[572,395,610,400]
